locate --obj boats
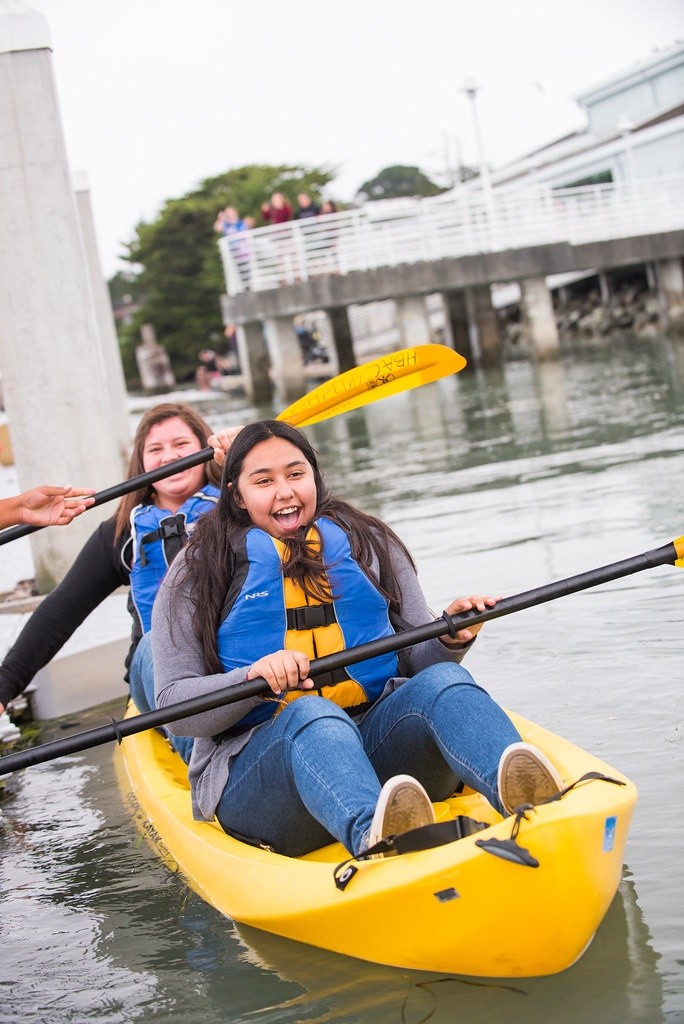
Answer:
[116,698,638,979]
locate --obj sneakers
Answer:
[498,742,564,816]
[369,775,435,860]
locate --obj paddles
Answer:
[1,530,684,783]
[0,344,468,551]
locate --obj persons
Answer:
[152,420,561,858]
[213,191,337,292]
[0,403,244,740]
[195,322,242,391]
[1,483,95,528]
[297,319,314,367]
